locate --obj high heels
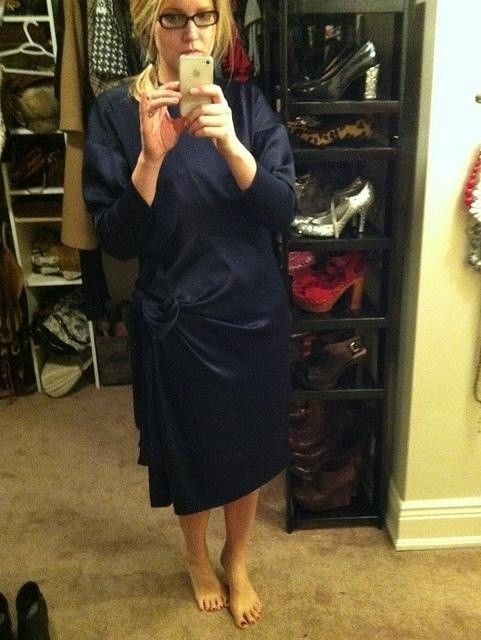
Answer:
[287,37,380,514]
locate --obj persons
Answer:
[82,0,299,630]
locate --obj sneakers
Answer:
[0,591,14,640]
[14,579,52,640]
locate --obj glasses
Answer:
[157,9,220,30]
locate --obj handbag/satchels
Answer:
[39,356,94,399]
[32,288,98,356]
[1,74,63,130]
[8,143,60,188]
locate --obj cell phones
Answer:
[179,56,214,117]
